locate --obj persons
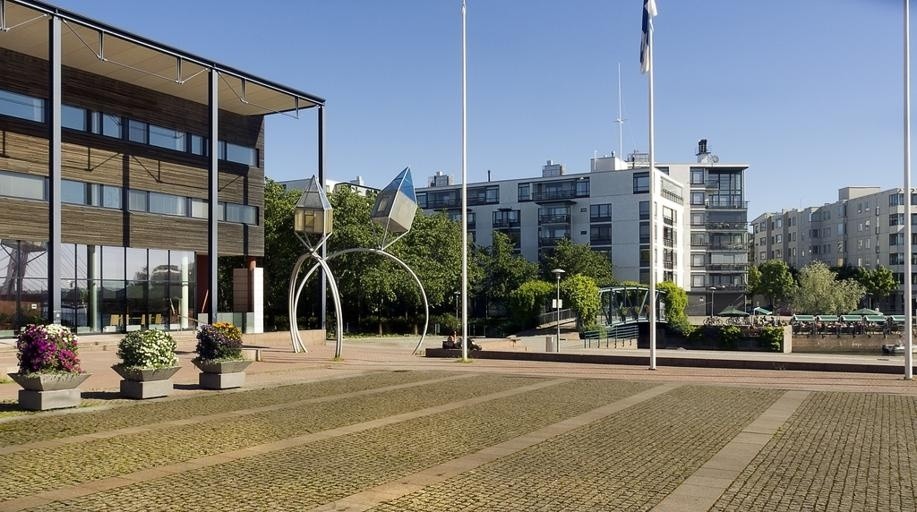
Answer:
[702,313,892,332]
[448,330,460,345]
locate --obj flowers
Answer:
[193,322,243,359]
[116,329,177,369]
[16,322,79,373]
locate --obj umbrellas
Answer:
[717,307,749,318]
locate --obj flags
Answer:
[639,0,659,80]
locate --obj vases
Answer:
[7,373,92,410]
[110,367,182,398]
[190,359,254,390]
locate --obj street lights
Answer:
[551,268,565,352]
[709,286,716,318]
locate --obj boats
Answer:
[882,343,917,354]
